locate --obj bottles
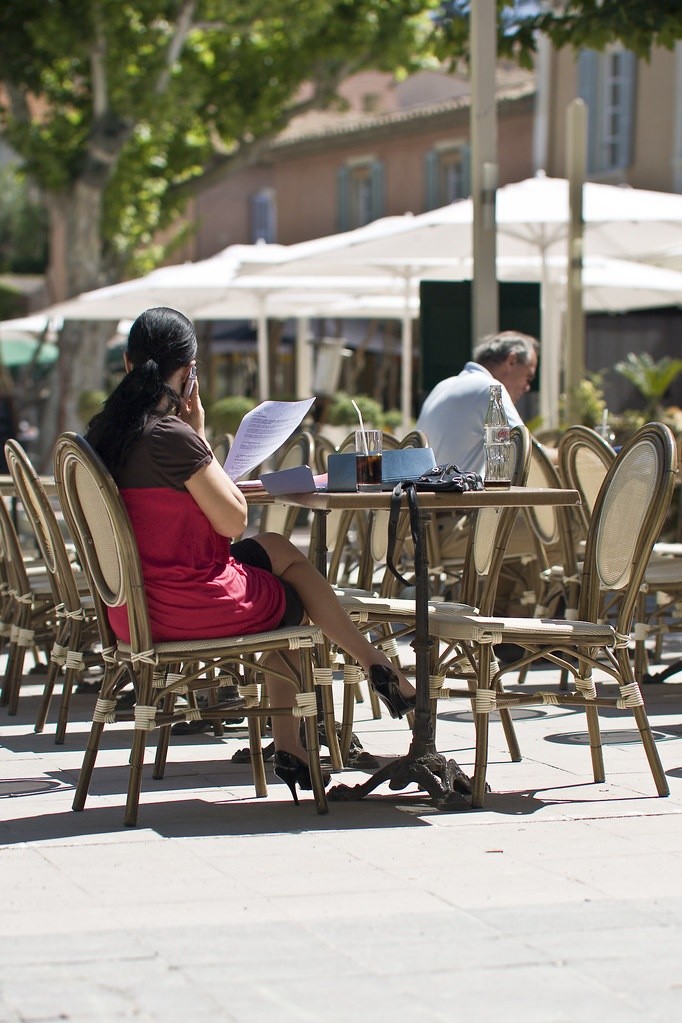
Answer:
[483,385,512,491]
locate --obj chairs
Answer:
[0,424,682,828]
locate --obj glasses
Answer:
[503,350,535,383]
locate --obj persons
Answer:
[81,307,416,806]
[415,331,558,664]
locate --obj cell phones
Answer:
[178,366,197,412]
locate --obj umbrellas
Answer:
[0,169,682,403]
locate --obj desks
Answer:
[274,486,582,812]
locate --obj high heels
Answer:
[368,664,416,720]
[273,750,331,806]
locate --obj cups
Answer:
[594,426,617,448]
[355,430,383,493]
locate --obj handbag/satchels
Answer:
[413,463,484,491]
